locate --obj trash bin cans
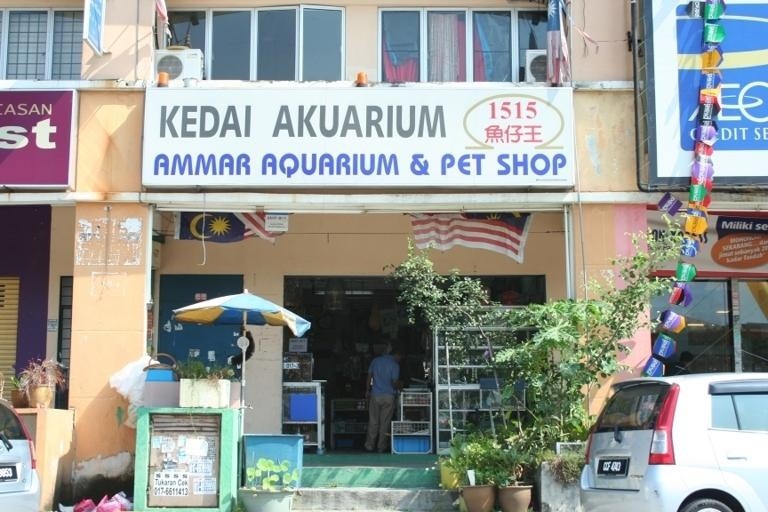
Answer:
[242,432,307,490]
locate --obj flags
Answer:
[410,212,534,264]
[172,213,284,247]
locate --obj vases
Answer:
[26,383,55,408]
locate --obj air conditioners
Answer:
[527,50,547,83]
[154,48,204,87]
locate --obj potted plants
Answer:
[179,357,236,408]
[239,458,300,512]
[440,433,534,512]
[10,376,27,408]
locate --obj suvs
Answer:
[578,372,768,512]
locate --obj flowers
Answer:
[20,356,67,401]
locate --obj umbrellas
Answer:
[171,289,311,489]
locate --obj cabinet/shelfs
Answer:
[284,382,433,455]
[434,306,530,456]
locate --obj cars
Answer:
[0,397,44,512]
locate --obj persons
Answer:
[671,350,694,375]
[363,337,404,453]
